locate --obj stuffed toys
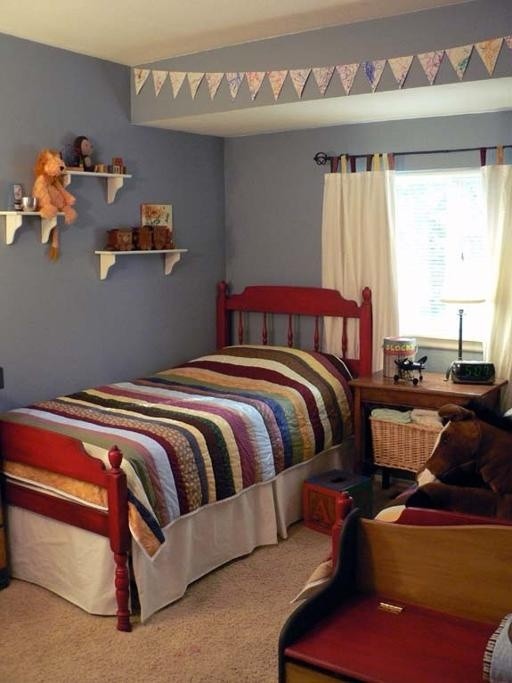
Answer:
[32,147,77,224]
[73,135,95,171]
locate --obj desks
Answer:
[348,368,508,466]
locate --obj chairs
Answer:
[276,507,511,682]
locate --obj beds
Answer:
[0,282,382,632]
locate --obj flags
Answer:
[266,69,288,101]
[205,72,224,100]
[224,72,245,99]
[360,59,386,93]
[152,70,168,96]
[134,67,151,96]
[312,65,335,96]
[335,63,359,96]
[187,71,205,99]
[417,49,444,85]
[289,68,311,99]
[474,37,504,76]
[245,71,266,100]
[445,43,474,81]
[169,70,187,99]
[387,55,414,89]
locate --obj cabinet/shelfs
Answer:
[58,168,133,204]
[0,210,69,246]
[94,247,190,281]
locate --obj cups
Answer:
[22,197,37,212]
